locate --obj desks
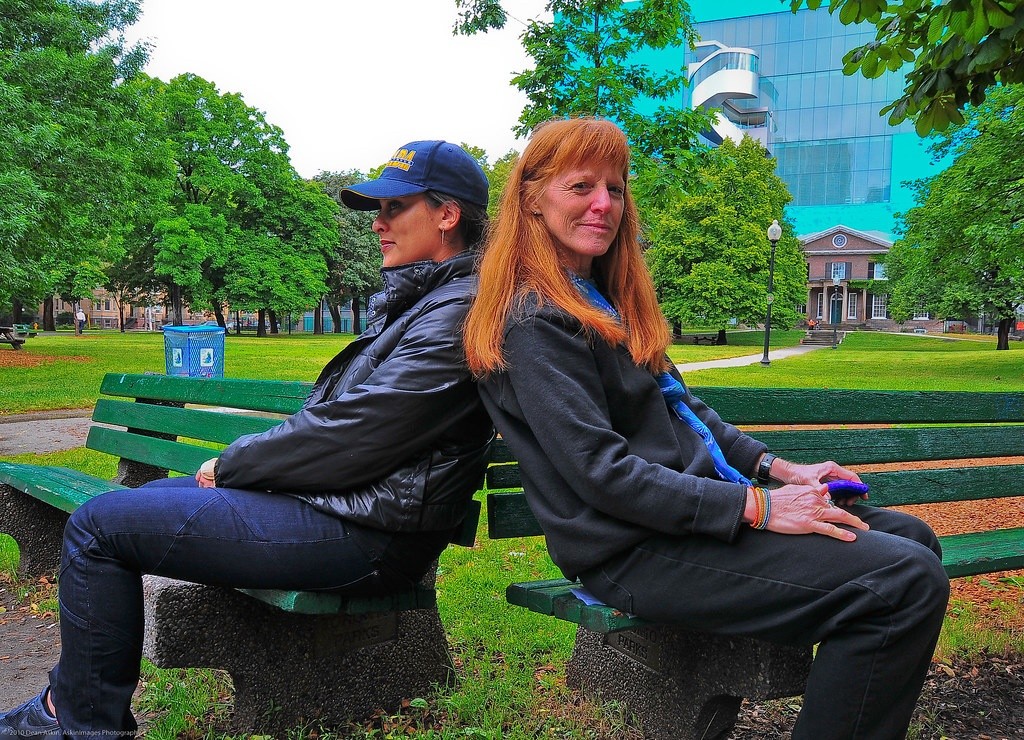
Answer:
[0,327,23,350]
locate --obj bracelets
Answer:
[750,486,772,530]
[756,453,777,485]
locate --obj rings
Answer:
[826,499,834,506]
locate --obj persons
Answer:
[463,117,949,740]
[0,142,496,740]
[76,309,86,333]
[815,320,820,330]
[808,318,814,330]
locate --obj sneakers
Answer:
[0,684,70,740]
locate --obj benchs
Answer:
[0,337,24,344]
[0,373,496,740]
[485,390,1024,740]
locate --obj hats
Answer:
[339,140,489,211]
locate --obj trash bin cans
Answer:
[161,324,226,378]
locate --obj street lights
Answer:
[761,219,783,365]
[829,273,843,348]
